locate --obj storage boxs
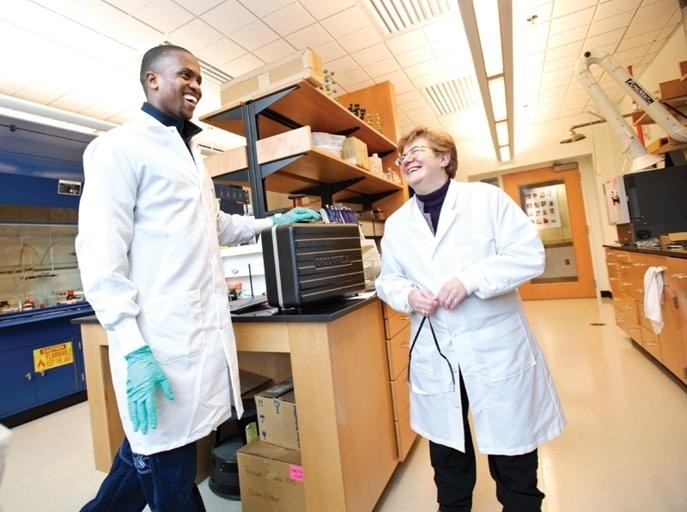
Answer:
[236,377,307,512]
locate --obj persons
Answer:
[71,45,321,511]
[375,126,562,512]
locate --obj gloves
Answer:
[272,207,321,224]
[124,345,174,434]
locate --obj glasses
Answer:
[395,145,425,166]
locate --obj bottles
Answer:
[66,290,77,305]
[373,207,386,222]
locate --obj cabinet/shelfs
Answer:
[606,248,687,386]
[1,339,79,416]
[384,298,415,464]
[198,78,401,237]
